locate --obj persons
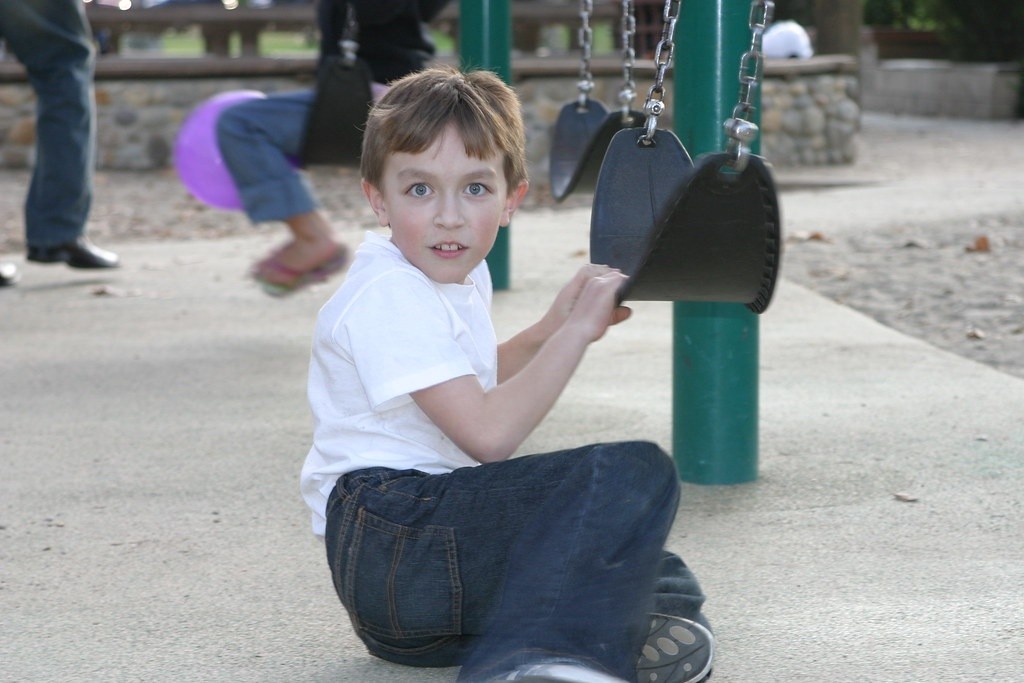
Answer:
[763,19,819,60]
[1,0,124,287]
[214,0,454,298]
[300,68,716,683]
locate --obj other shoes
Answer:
[631,612,718,683]
[482,647,634,683]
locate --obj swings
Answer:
[300,1,375,166]
[589,0,777,314]
[546,1,649,203]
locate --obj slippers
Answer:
[253,241,346,294]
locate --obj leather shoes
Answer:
[27,237,119,267]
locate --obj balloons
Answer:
[174,91,268,212]
